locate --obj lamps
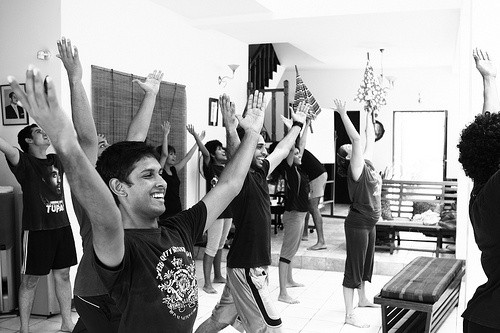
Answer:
[218,63,239,87]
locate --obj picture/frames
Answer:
[0,83,29,126]
[222,102,232,127]
[208,97,219,127]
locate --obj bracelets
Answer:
[292,120,304,130]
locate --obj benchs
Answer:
[373,256,466,333]
[376,180,458,257]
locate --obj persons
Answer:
[193,93,310,333]
[186,123,234,294]
[7,61,265,333]
[6,91,24,118]
[330,98,382,329]
[36,159,63,207]
[0,122,107,333]
[277,113,310,303]
[55,35,164,333]
[456,47,500,333]
[268,140,329,250]
[155,120,206,221]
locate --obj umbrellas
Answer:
[292,64,322,134]
[353,51,387,124]
